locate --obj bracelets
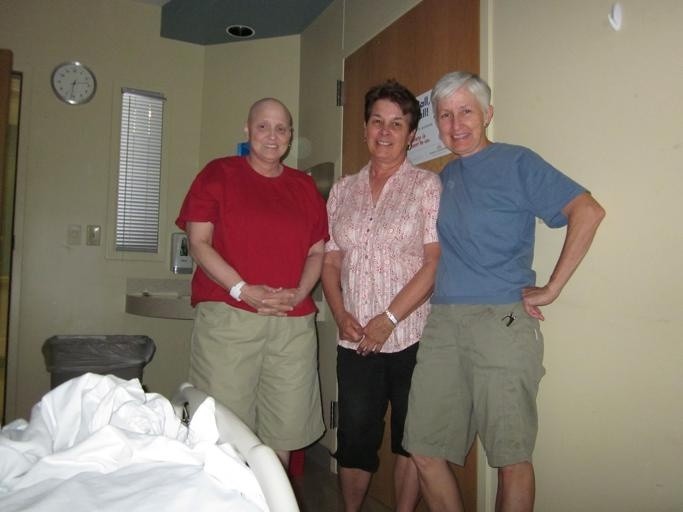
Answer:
[230,280,246,302]
[385,309,398,327]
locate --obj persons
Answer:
[402,71,606,512]
[175,98,330,471]
[320,77,443,512]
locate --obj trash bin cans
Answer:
[40,333,157,390]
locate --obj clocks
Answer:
[50,59,97,106]
